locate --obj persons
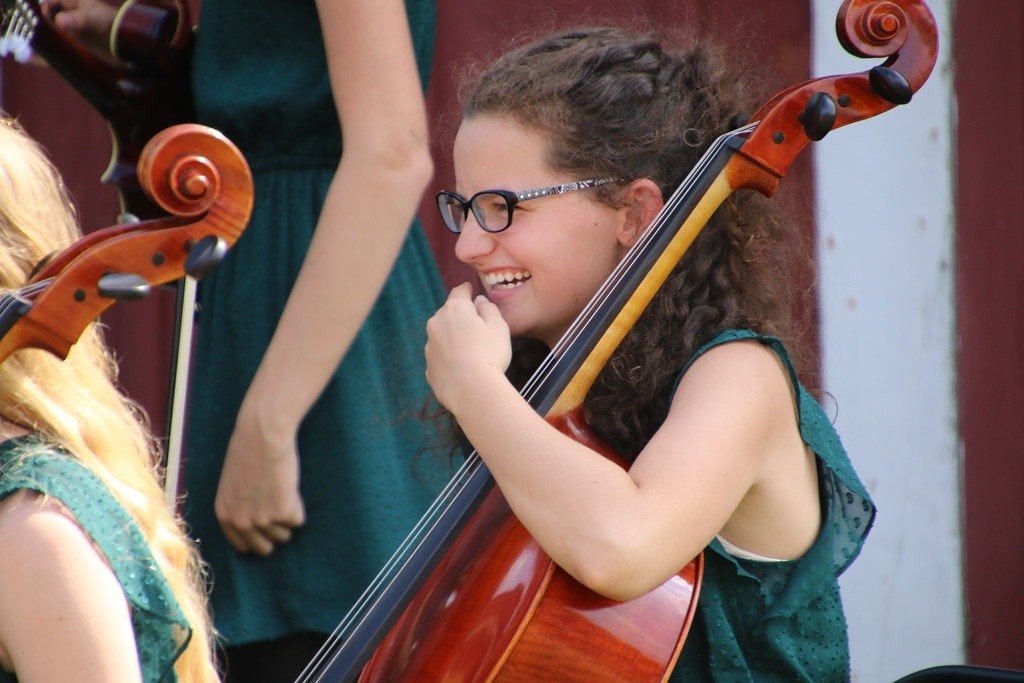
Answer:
[28,2,440,683]
[421,25,853,682]
[3,110,222,683]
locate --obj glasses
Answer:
[436,175,627,235]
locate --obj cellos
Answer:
[291,1,940,683]
[1,122,256,366]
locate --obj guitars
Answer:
[0,1,206,221]
